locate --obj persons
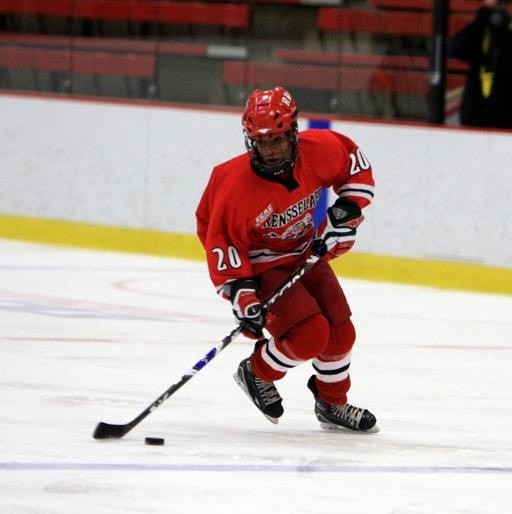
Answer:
[440,0,511,130]
[194,84,378,433]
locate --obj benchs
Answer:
[0,2,482,115]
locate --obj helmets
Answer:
[241,86,299,177]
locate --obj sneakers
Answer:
[238,353,284,418]
[315,396,376,431]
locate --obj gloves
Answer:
[314,207,366,263]
[222,274,276,341]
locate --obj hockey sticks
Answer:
[93,256,318,439]
